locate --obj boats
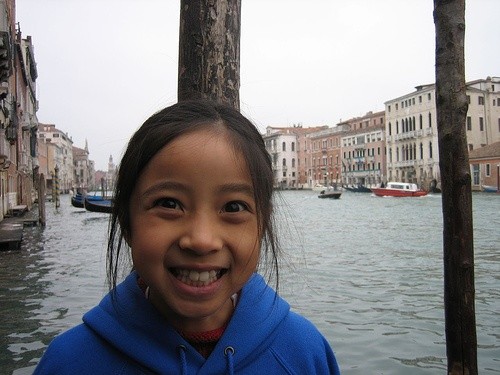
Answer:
[481,185,497,193]
[318,189,341,199]
[374,182,427,197]
[71,192,114,213]
[343,182,385,192]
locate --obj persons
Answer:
[33,101,339,375]
